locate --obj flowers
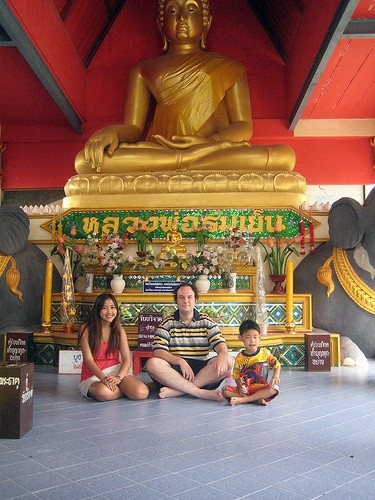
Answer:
[299,219,306,255]
[253,220,304,275]
[51,212,252,277]
[309,220,315,251]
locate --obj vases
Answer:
[136,252,148,258]
[269,274,287,295]
[110,274,126,294]
[228,273,237,293]
[85,273,94,293]
[195,274,211,295]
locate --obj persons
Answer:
[145,283,232,401]
[79,293,149,401]
[74,0,296,172]
[222,320,281,407]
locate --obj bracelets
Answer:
[115,375,122,380]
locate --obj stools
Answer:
[133,350,153,376]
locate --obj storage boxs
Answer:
[58,349,83,375]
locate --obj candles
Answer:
[43,258,53,323]
[286,258,294,323]
